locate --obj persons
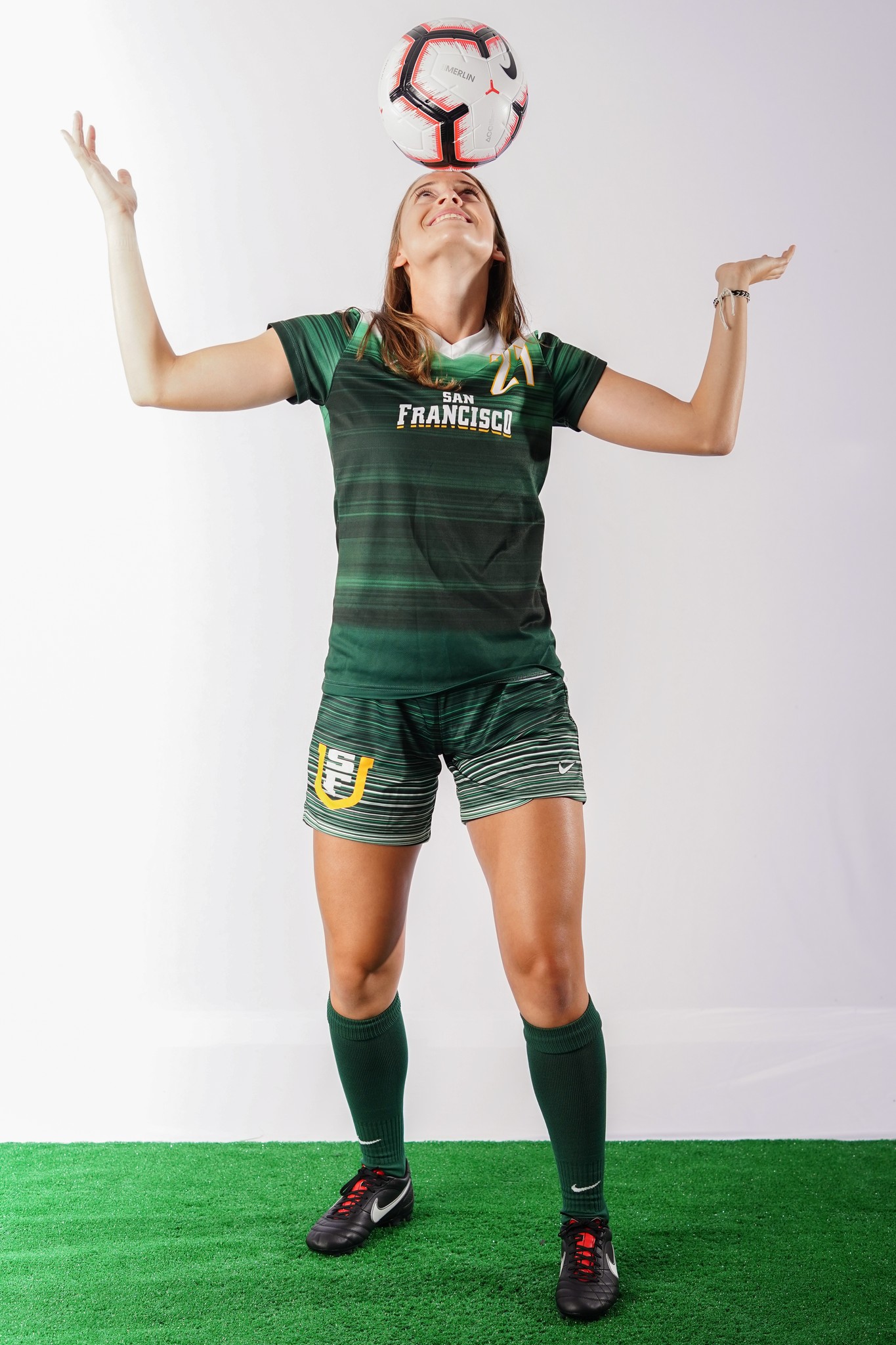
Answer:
[54,89,796,1322]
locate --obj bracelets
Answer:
[713,287,749,330]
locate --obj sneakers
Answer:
[305,1158,415,1255]
[554,1212,621,1320]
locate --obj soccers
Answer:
[377,17,529,171]
